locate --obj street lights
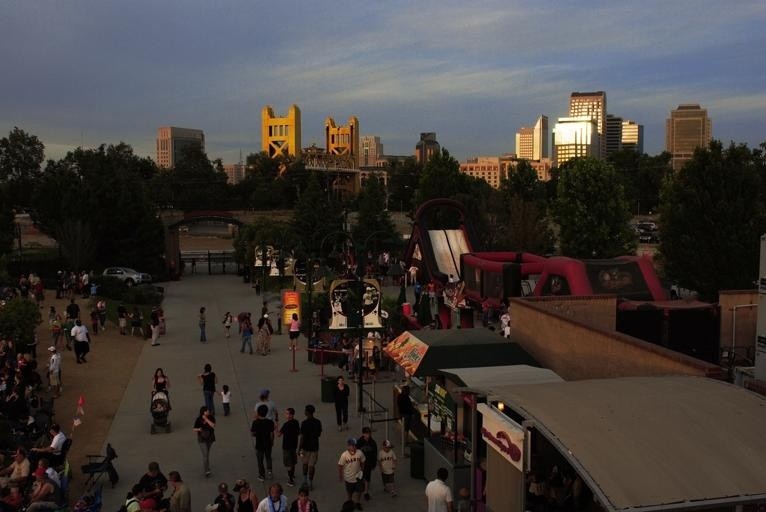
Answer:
[404,185,414,192]
[310,228,407,417]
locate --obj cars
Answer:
[630,220,658,243]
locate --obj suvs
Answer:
[103,267,152,288]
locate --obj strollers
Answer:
[149,389,172,434]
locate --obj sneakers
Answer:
[45,387,62,399]
[76,357,87,364]
[255,467,320,491]
[385,487,401,497]
[201,471,212,479]
[355,492,372,511]
[337,422,350,433]
[199,334,298,357]
[92,326,160,347]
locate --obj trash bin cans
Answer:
[321,376,338,402]
[410,440,426,480]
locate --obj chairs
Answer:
[80,444,119,489]
[56,439,72,489]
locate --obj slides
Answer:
[416,225,474,284]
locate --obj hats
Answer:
[48,346,56,352]
[260,389,270,399]
[346,437,359,446]
[216,478,250,493]
[382,439,394,450]
